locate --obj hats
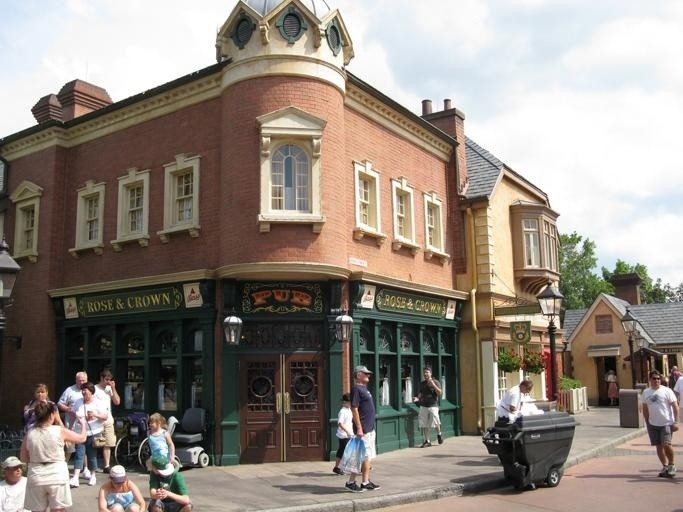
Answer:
[343,395,351,401]
[110,465,126,483]
[353,366,372,374]
[150,454,174,476]
[2,456,26,471]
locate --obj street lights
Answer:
[620,306,646,389]
[537,275,565,401]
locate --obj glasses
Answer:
[653,378,660,380]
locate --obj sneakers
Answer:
[438,433,443,444]
[83,470,91,479]
[333,467,344,475]
[361,481,381,491]
[668,465,676,476]
[421,440,431,448]
[659,465,668,477]
[88,476,96,486]
[103,466,111,473]
[345,481,364,493]
[70,477,79,487]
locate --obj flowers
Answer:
[521,348,547,373]
[498,347,521,373]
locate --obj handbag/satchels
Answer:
[338,435,366,474]
[92,437,107,448]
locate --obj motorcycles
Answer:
[171,407,209,467]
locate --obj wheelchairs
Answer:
[114,413,151,472]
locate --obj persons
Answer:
[19,400,87,512]
[149,454,193,512]
[68,382,108,486]
[671,365,678,389]
[605,370,618,405]
[23,383,65,435]
[673,374,683,422]
[58,372,88,462]
[642,370,680,477]
[344,365,381,493]
[132,383,142,408]
[149,412,176,464]
[333,393,373,476]
[99,464,145,512]
[0,455,28,512]
[93,370,120,467]
[415,368,443,447]
[497,379,534,424]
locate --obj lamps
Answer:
[223,304,243,346]
[335,300,354,343]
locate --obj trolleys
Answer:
[482,412,575,489]
